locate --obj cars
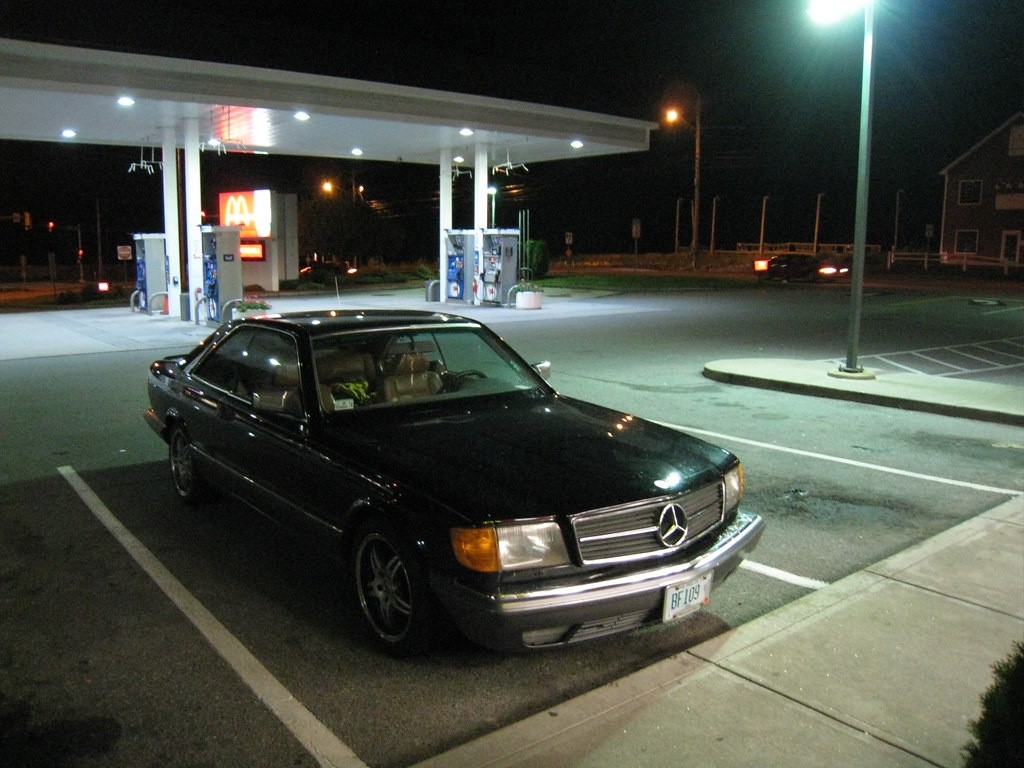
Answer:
[141,309,767,657]
[755,253,820,284]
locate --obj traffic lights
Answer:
[77,249,84,265]
[47,220,55,232]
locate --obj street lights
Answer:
[488,186,497,228]
[665,94,703,259]
[805,0,880,382]
